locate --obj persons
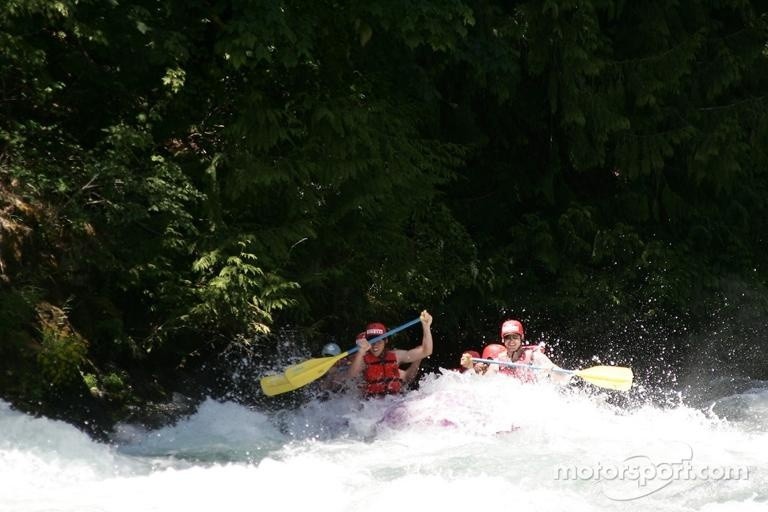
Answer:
[447,350,482,376]
[346,308,434,400]
[462,343,508,377]
[316,342,352,401]
[393,356,423,386]
[484,320,582,386]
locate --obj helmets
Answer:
[482,345,505,359]
[356,323,388,344]
[322,344,341,357]
[502,319,523,342]
[468,351,480,357]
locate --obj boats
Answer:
[377,396,527,443]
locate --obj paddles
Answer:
[260,372,297,396]
[285,317,424,388]
[472,357,633,391]
